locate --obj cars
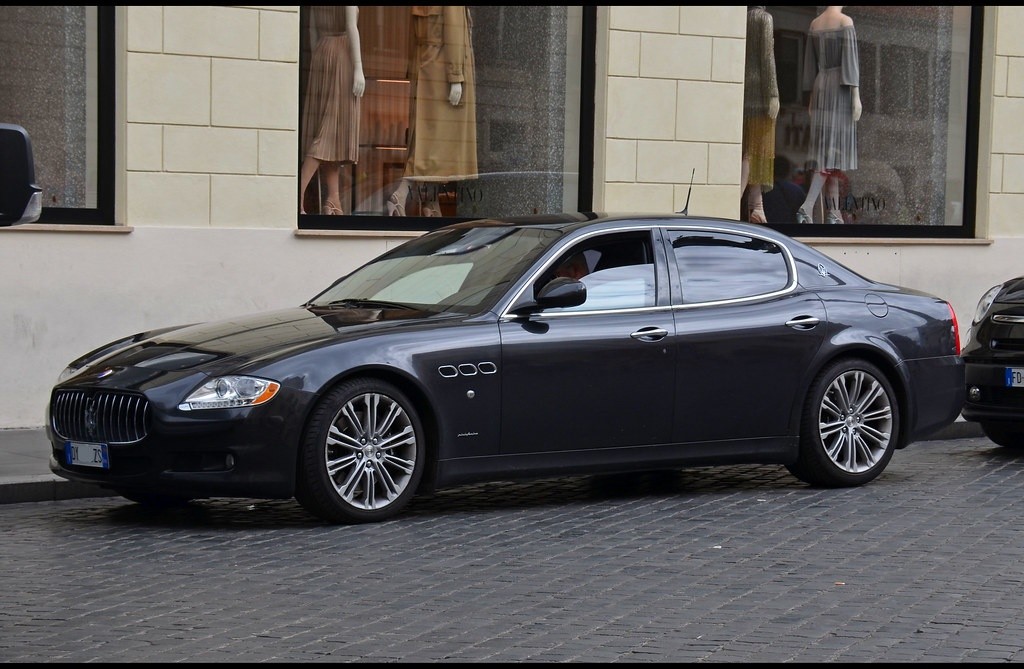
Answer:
[44,210,965,522]
[961,277,1024,458]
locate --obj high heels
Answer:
[387,194,406,216]
[796,206,813,224]
[423,202,442,217]
[323,200,343,215]
[826,212,844,224]
[750,210,768,223]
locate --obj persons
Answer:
[759,155,818,224]
[386,5,479,217]
[795,6,863,224]
[300,6,366,215]
[740,6,781,224]
